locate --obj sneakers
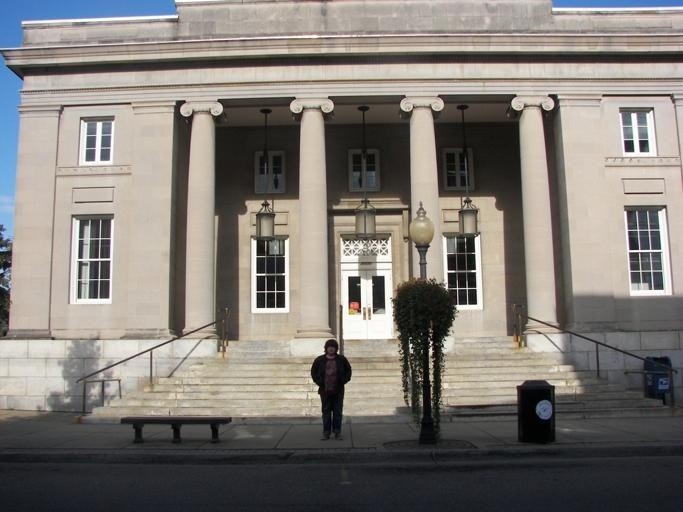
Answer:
[319,431,332,440]
[335,433,344,441]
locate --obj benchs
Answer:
[120,417,232,443]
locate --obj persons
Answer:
[310,339,352,441]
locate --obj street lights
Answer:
[410,202,434,444]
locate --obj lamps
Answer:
[458,104,479,238]
[354,105,376,239]
[255,108,276,242]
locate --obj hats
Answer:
[324,339,339,350]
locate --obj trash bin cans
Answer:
[517,380,555,444]
[643,356,672,405]
